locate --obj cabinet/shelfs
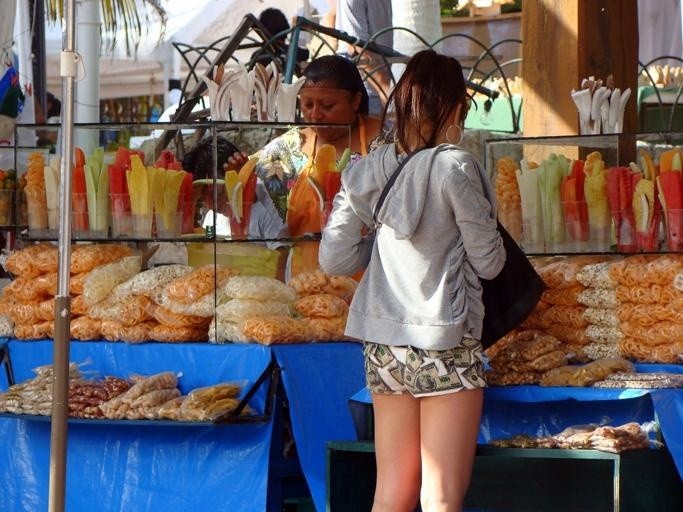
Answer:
[1,117,682,512]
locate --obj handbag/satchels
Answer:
[480,219,544,353]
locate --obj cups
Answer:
[495,206,679,251]
[23,189,184,244]
[576,107,623,134]
[208,91,297,122]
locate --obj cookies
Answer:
[0,244,683,421]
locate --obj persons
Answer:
[150,89,213,136]
[32,91,62,144]
[181,134,293,286]
[221,55,388,282]
[317,49,506,510]
